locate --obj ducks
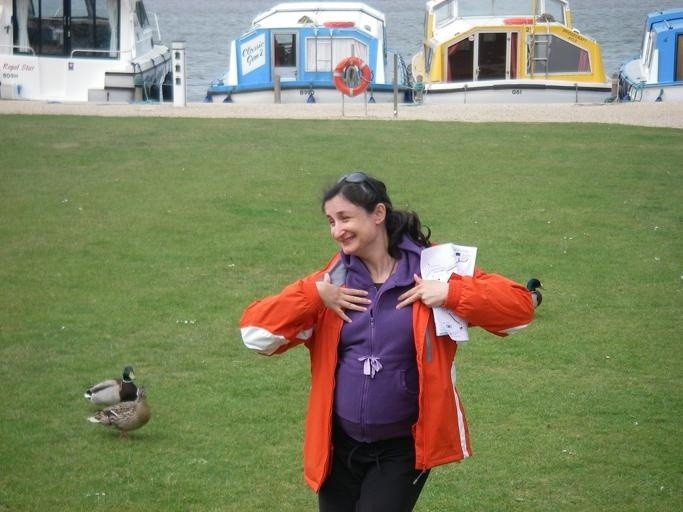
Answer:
[84,385,151,440]
[84,366,137,407]
[527,278,547,310]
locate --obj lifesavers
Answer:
[333,57,371,97]
[324,21,355,27]
[503,18,534,24]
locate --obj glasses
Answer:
[332,172,381,196]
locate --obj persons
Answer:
[238,172,534,511]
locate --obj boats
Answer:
[615,6,682,104]
[0,0,172,104]
[406,0,614,104]
[205,1,409,104]
